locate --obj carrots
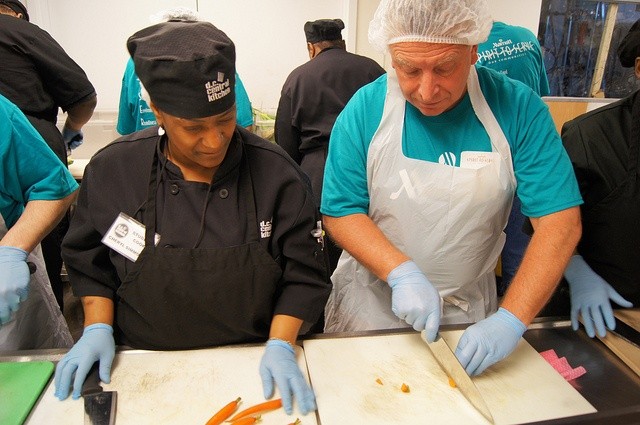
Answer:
[230,414,262,425]
[287,417,302,425]
[204,397,242,425]
[225,398,284,422]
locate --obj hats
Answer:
[0,0,29,22]
[304,19,344,43]
[127,20,236,119]
[618,20,639,65]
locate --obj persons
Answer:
[116,8,254,136]
[53,17,333,416]
[273,18,387,271]
[0,93,81,356]
[0,0,97,311]
[477,21,551,295]
[522,20,640,339]
[319,0,584,379]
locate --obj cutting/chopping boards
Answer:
[303,329,599,421]
[0,361,54,421]
[27,343,317,421]
[577,309,640,379]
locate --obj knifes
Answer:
[81,360,117,425]
[420,330,495,425]
[601,313,640,348]
